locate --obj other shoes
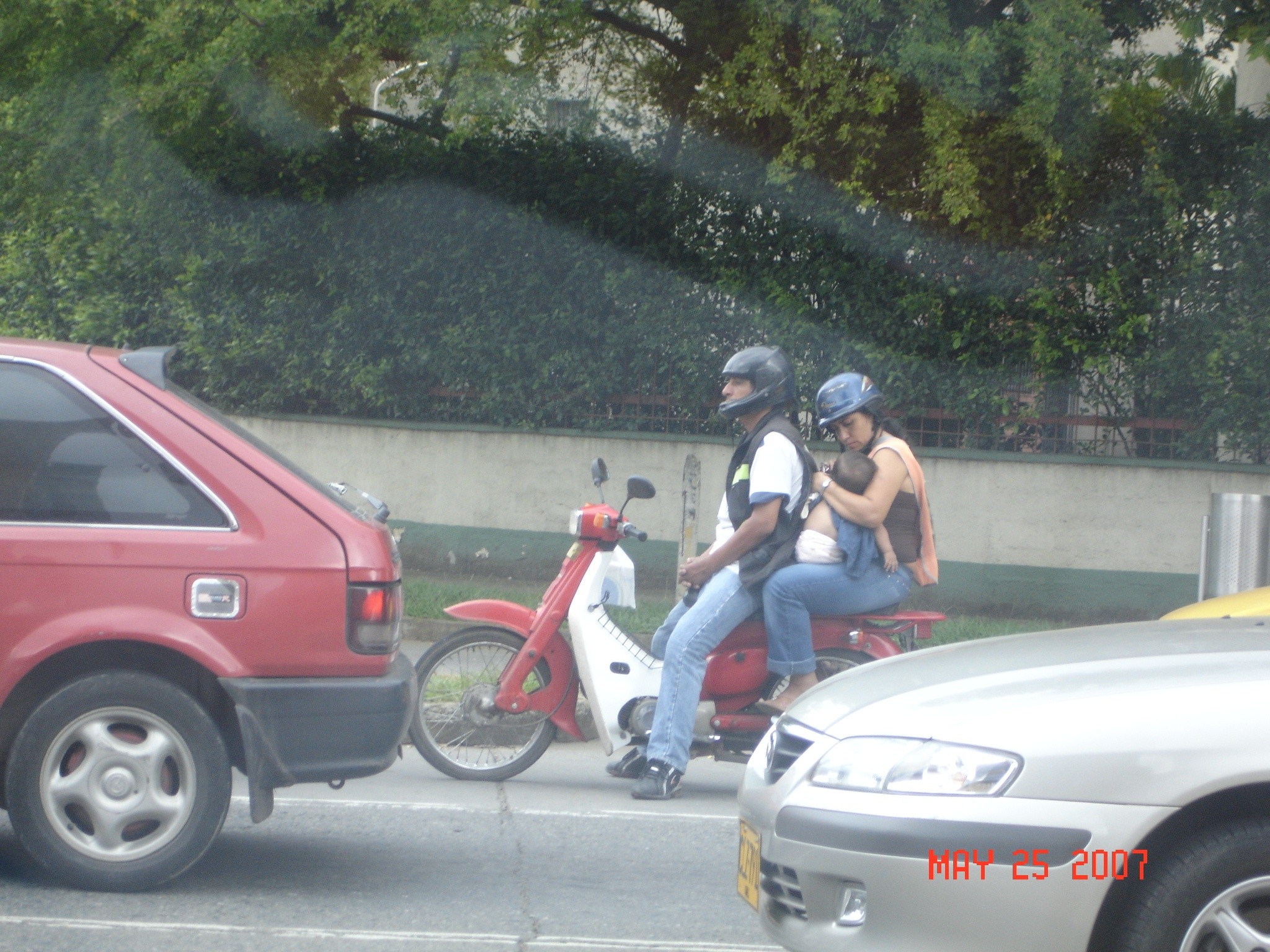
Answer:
[755,693,794,716]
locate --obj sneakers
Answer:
[606,748,648,778]
[631,756,682,800]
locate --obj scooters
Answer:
[408,458,948,781]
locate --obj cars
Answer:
[0,336,423,891]
[738,587,1270,952]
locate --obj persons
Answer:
[631,345,819,799]
[796,451,898,573]
[755,372,939,715]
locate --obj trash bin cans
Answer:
[1207,492,1269,599]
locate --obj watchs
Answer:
[819,478,833,496]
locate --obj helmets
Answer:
[718,345,797,418]
[816,372,885,433]
